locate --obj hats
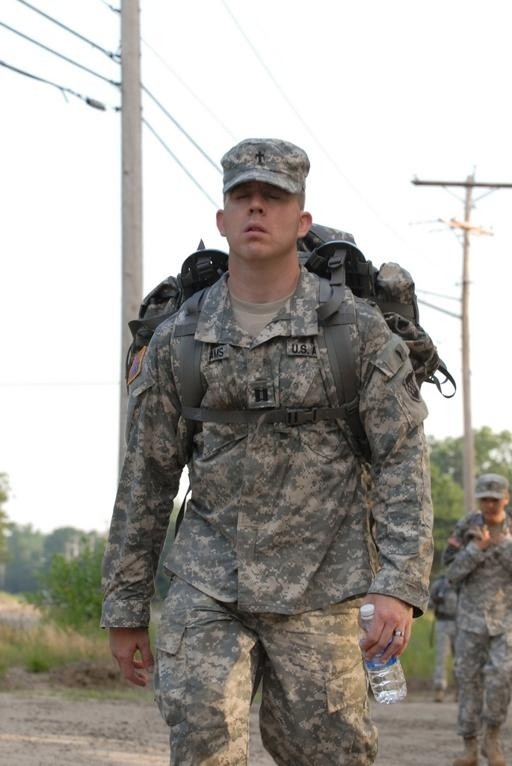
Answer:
[474,473,509,499]
[221,138,309,195]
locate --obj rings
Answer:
[394,631,403,639]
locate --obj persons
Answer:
[99,138,434,764]
[444,472,512,766]
[429,576,462,703]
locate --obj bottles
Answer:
[357,601,408,705]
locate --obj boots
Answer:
[481,724,506,766]
[452,735,478,766]
[433,688,445,701]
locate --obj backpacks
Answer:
[127,223,457,461]
